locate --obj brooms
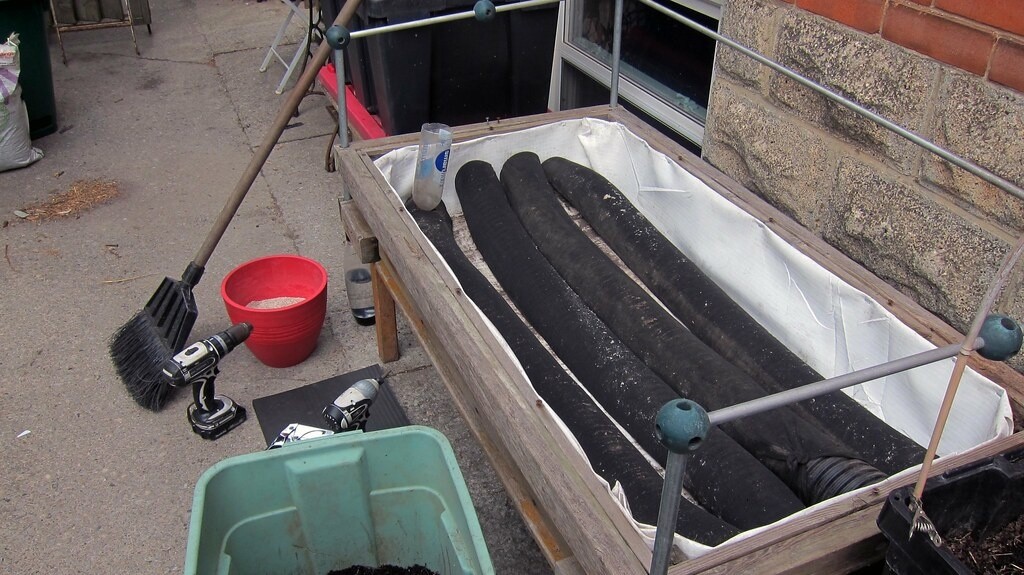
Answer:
[106,0,364,413]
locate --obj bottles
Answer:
[343,231,377,326]
[414,122,453,212]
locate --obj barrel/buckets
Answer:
[221,255,327,367]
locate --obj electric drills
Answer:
[161,322,255,439]
[322,367,393,431]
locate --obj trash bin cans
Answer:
[877,443,1024,575]
[184,423,496,575]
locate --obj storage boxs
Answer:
[182,425,495,575]
[320,0,559,140]
